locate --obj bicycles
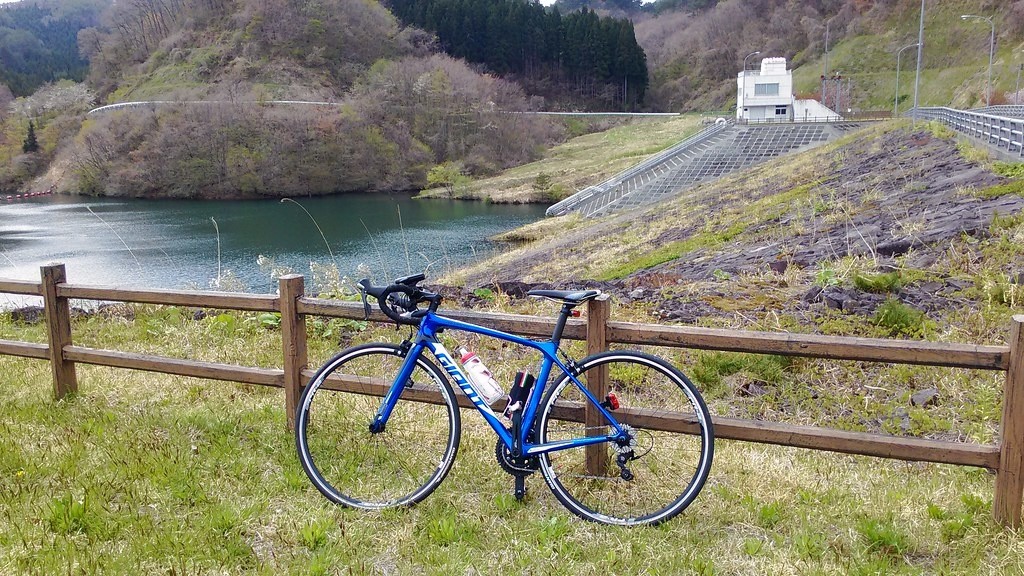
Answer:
[293,274,713,533]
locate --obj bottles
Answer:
[503,370,535,421]
[458,347,504,405]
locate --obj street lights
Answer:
[893,42,923,116]
[740,50,761,124]
[961,13,994,107]
